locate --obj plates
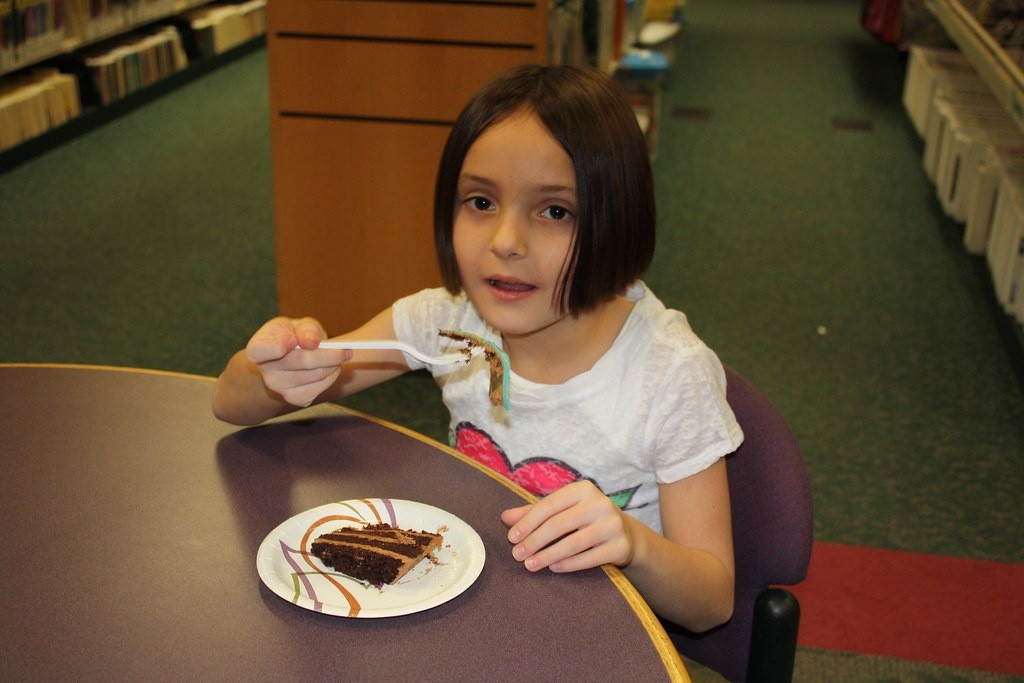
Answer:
[256,499,487,620]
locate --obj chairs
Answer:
[642,359,813,683]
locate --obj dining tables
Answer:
[0,360,694,682]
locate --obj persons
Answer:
[209,61,741,632]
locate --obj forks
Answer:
[293,341,485,367]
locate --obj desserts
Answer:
[308,328,515,593]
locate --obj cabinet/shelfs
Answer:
[0,1,267,177]
[267,0,682,339]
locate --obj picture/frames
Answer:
[922,0,1024,133]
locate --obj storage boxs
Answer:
[900,43,1023,330]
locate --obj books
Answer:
[538,0,682,72]
[0,0,269,169]
[895,36,1024,327]
